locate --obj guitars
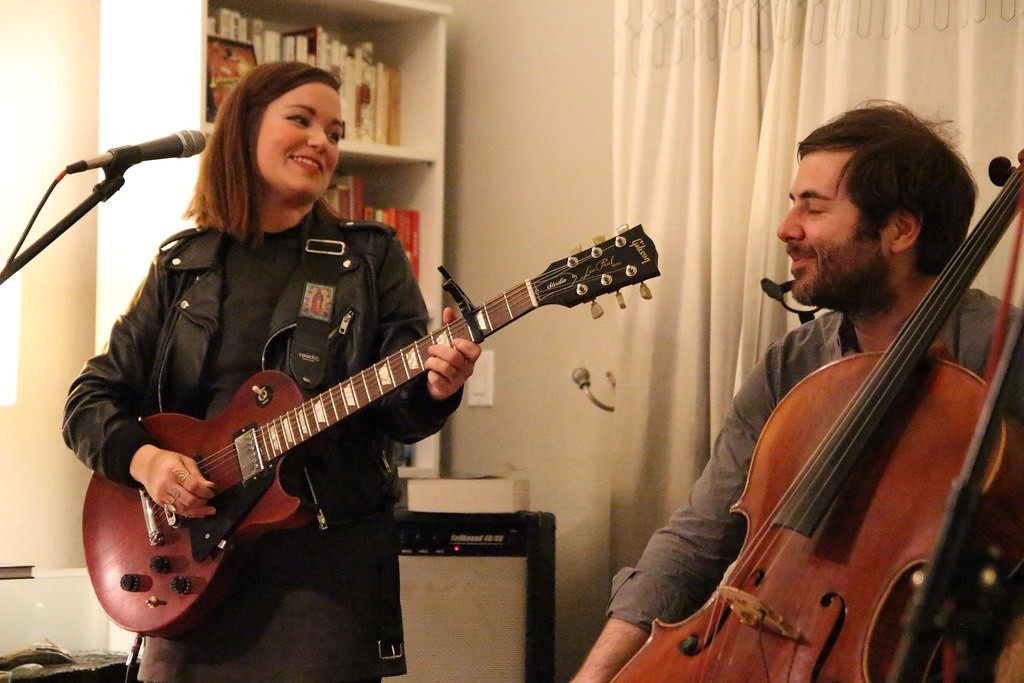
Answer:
[82,221,664,640]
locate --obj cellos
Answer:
[610,147,1024,682]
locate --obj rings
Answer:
[170,498,176,504]
[170,469,189,485]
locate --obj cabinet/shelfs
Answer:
[95,0,454,478]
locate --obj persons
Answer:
[61,61,482,683]
[573,108,1024,683]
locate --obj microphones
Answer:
[67,129,206,174]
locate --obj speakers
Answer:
[381,509,558,683]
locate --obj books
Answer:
[206,7,400,147]
[324,176,419,283]
